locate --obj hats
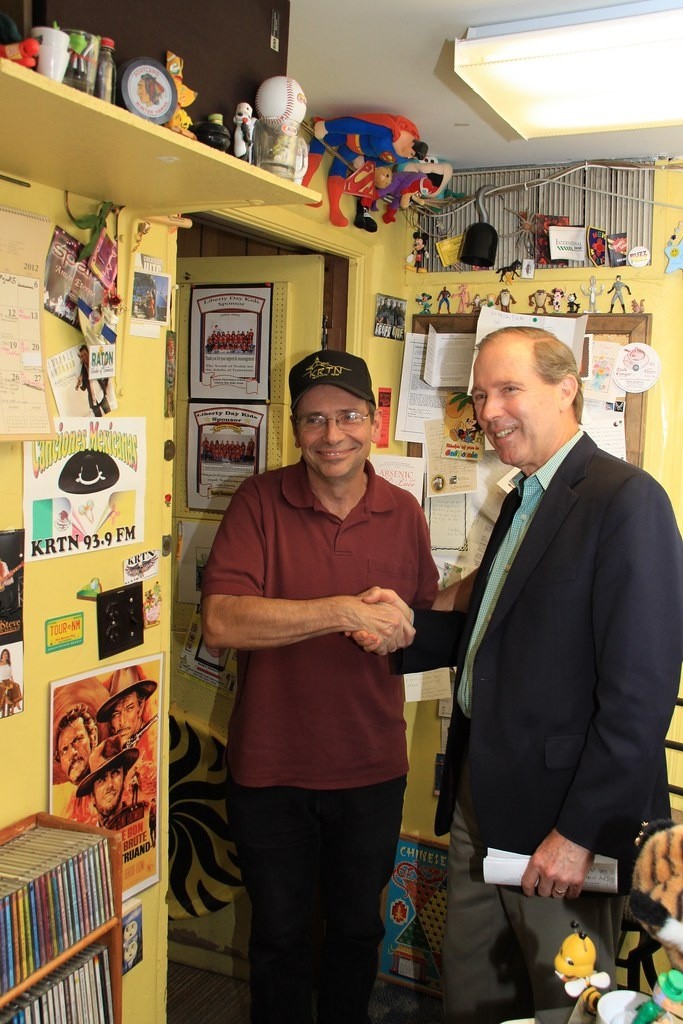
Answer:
[289,350,376,415]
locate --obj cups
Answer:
[60,28,101,96]
[253,120,308,182]
[31,27,70,84]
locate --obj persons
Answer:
[607,275,631,313]
[437,286,451,314]
[207,324,255,352]
[55,666,157,846]
[78,345,112,416]
[200,350,480,1024]
[346,325,683,1024]
[376,298,405,326]
[202,437,255,461]
[525,264,530,275]
[0,649,22,716]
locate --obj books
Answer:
[0,943,114,1024]
[0,827,114,996]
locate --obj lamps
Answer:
[454,1,682,140]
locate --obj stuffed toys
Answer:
[302,112,466,232]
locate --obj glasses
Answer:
[293,410,370,431]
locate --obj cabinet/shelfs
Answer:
[0,810,124,1024]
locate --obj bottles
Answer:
[92,38,116,106]
